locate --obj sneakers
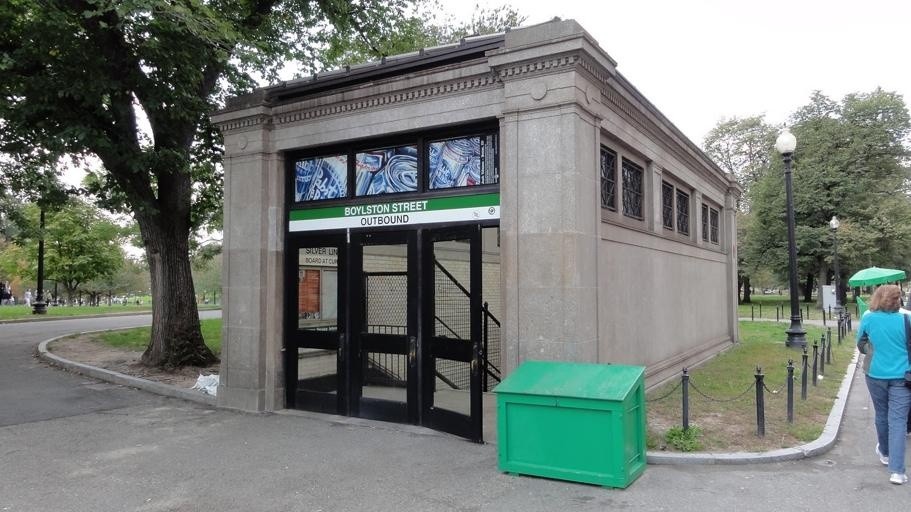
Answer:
[890,472,908,484]
[876,443,889,465]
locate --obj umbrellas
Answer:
[848,266,907,294]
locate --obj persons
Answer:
[23,288,53,308]
[854,282,911,487]
[858,304,910,435]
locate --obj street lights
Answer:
[32,165,47,315]
[777,122,807,316]
[829,215,844,305]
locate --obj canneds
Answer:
[295,136,485,203]
